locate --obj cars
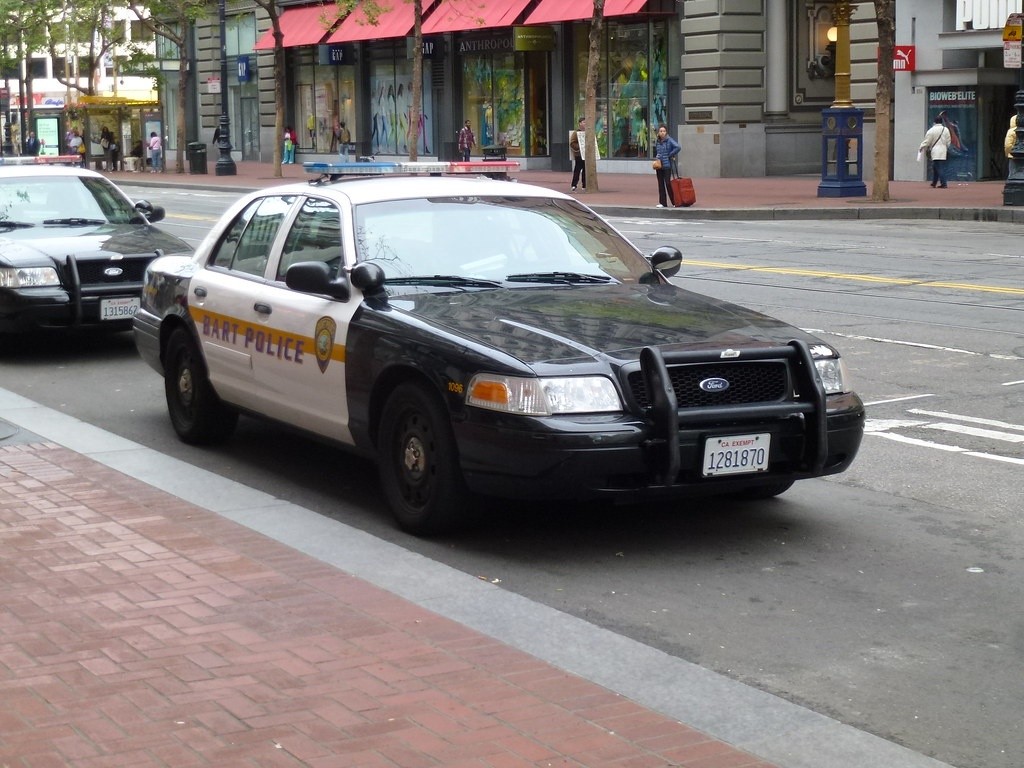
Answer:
[0,154,204,351]
[140,166,868,546]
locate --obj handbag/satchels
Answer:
[917,149,923,161]
[653,159,661,169]
[149,147,153,150]
[110,144,116,149]
[79,143,85,154]
[926,148,931,159]
[101,138,109,148]
[287,138,292,151]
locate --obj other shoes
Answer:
[931,183,936,188]
[582,188,586,191]
[289,161,293,163]
[656,203,664,208]
[134,170,141,172]
[938,185,947,188]
[282,162,285,164]
[572,186,575,191]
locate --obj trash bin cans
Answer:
[187,142,207,175]
[482,144,507,181]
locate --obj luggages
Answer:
[669,155,696,207]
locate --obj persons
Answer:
[481,101,494,145]
[281,127,297,164]
[66,127,86,168]
[371,82,431,154]
[919,115,952,188]
[656,124,681,207]
[100,126,118,171]
[570,118,586,191]
[150,132,162,173]
[212,126,220,147]
[129,140,143,158]
[337,122,350,163]
[306,111,315,137]
[1000,115,1017,193]
[458,120,476,162]
[27,132,41,156]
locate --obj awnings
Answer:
[325,0,436,44]
[252,2,353,50]
[523,0,676,26]
[420,0,532,34]
